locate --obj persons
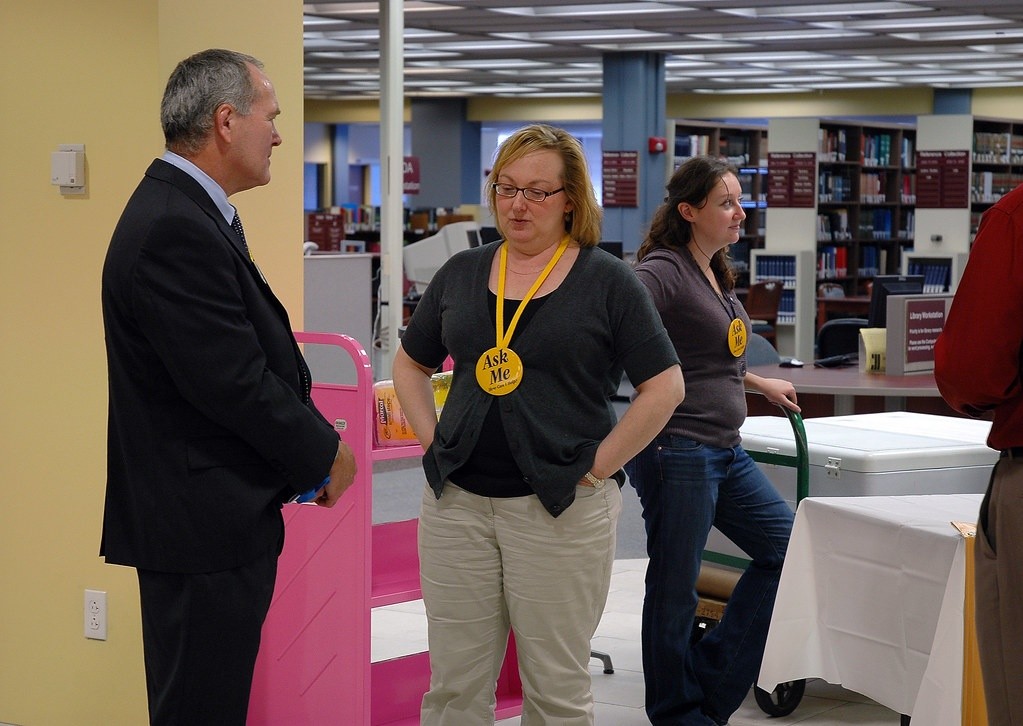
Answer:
[623,158,795,725]
[98,48,360,726]
[393,125,685,726]
[932,185,1023,726]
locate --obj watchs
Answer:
[585,471,607,489]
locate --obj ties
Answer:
[230,206,312,411]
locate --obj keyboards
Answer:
[814,353,858,368]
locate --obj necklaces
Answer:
[506,267,545,275]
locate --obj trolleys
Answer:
[695,390,806,717]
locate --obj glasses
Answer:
[491,176,565,203]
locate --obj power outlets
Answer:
[83,587,107,642]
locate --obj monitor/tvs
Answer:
[401,222,483,294]
[867,275,926,329]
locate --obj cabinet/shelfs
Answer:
[747,112,915,370]
[667,120,772,284]
[244,329,526,726]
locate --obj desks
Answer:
[754,492,988,726]
[913,113,1021,292]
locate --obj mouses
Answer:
[779,358,804,368]
